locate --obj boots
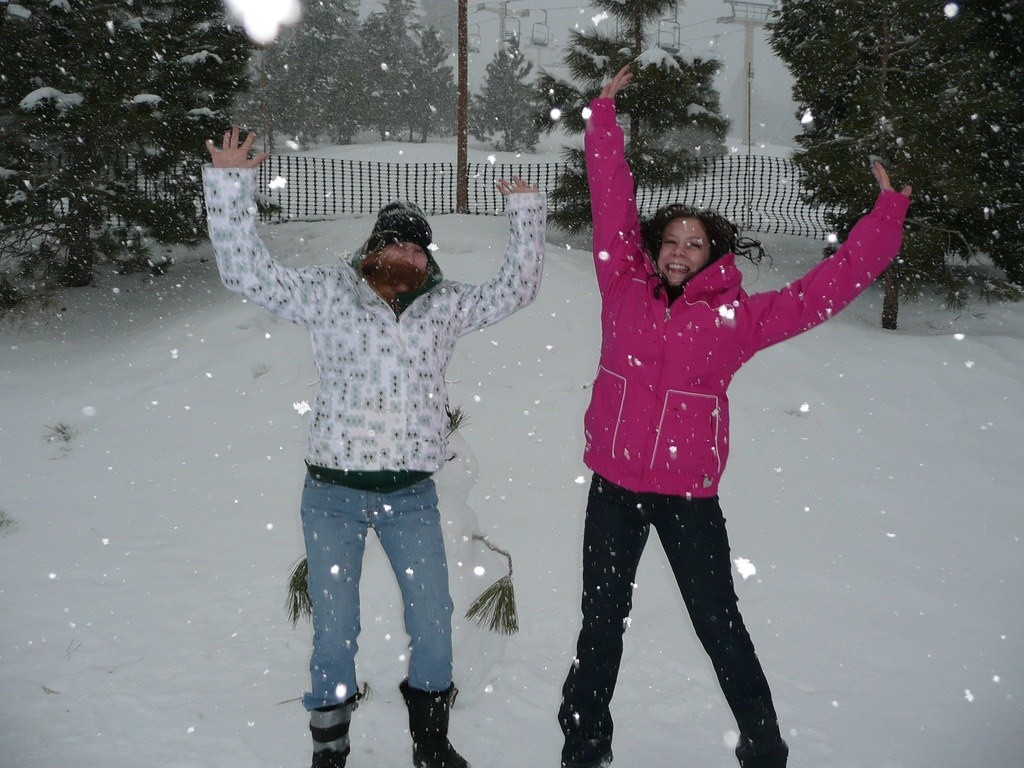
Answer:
[309,685,362,768]
[398,678,472,768]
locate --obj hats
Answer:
[367,201,432,253]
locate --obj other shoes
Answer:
[558,713,614,768]
[736,734,789,768]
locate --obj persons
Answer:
[201,126,547,768]
[556,66,913,768]
